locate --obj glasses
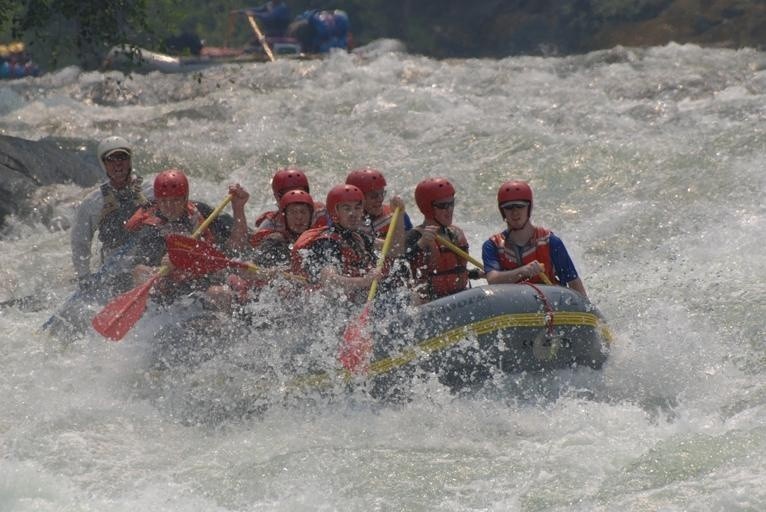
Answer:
[432,200,454,209]
[369,190,387,199]
[504,204,525,210]
[108,154,130,162]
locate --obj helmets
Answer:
[154,170,189,198]
[415,177,455,219]
[346,170,386,203]
[497,180,533,220]
[279,190,314,225]
[326,184,365,224]
[272,170,309,201]
[97,136,132,173]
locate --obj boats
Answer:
[100,39,407,75]
[44,283,610,425]
[0,58,42,78]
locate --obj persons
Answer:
[259,163,329,230]
[158,2,356,72]
[400,176,485,305]
[0,42,37,81]
[345,167,411,241]
[71,135,156,292]
[205,189,315,314]
[292,182,405,320]
[121,167,251,296]
[482,181,586,299]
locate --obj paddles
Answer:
[93,191,237,341]
[166,233,311,285]
[338,207,400,375]
